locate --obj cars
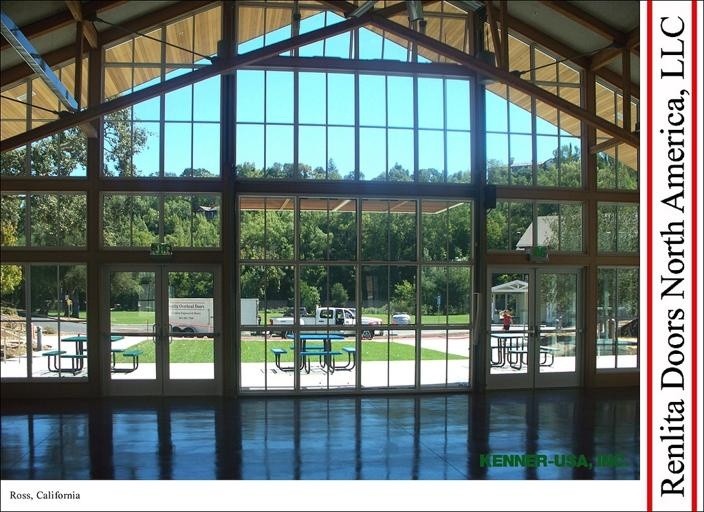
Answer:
[391,312,410,325]
[282,306,307,318]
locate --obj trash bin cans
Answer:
[393,314,410,325]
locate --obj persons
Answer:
[503,310,513,330]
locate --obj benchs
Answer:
[487,328,557,371]
[39,332,144,376]
[269,331,359,373]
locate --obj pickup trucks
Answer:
[269,306,383,340]
